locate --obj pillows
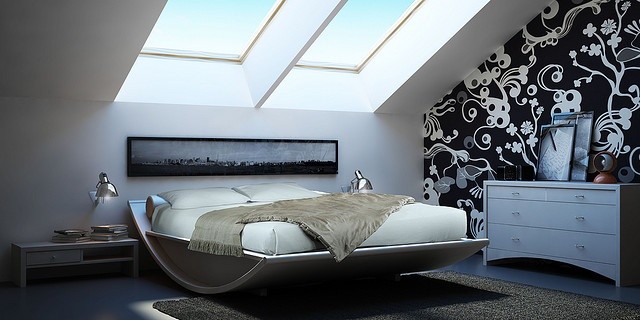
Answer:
[156,187,250,210]
[232,182,323,203]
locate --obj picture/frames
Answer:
[534,124,577,182]
[551,111,595,182]
[126,136,338,177]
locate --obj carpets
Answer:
[152,271,640,320]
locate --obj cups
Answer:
[341,186,351,192]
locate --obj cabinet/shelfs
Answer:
[483,181,640,287]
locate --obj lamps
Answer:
[341,170,373,193]
[89,171,119,207]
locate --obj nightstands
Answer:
[10,238,138,287]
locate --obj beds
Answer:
[127,189,490,294]
